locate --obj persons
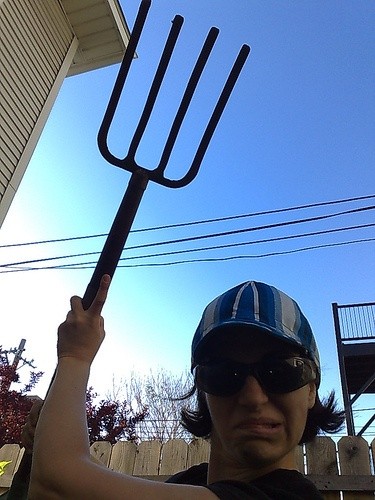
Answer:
[18,272,349,499]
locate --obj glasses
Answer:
[194,353,319,396]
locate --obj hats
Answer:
[191,281,322,375]
